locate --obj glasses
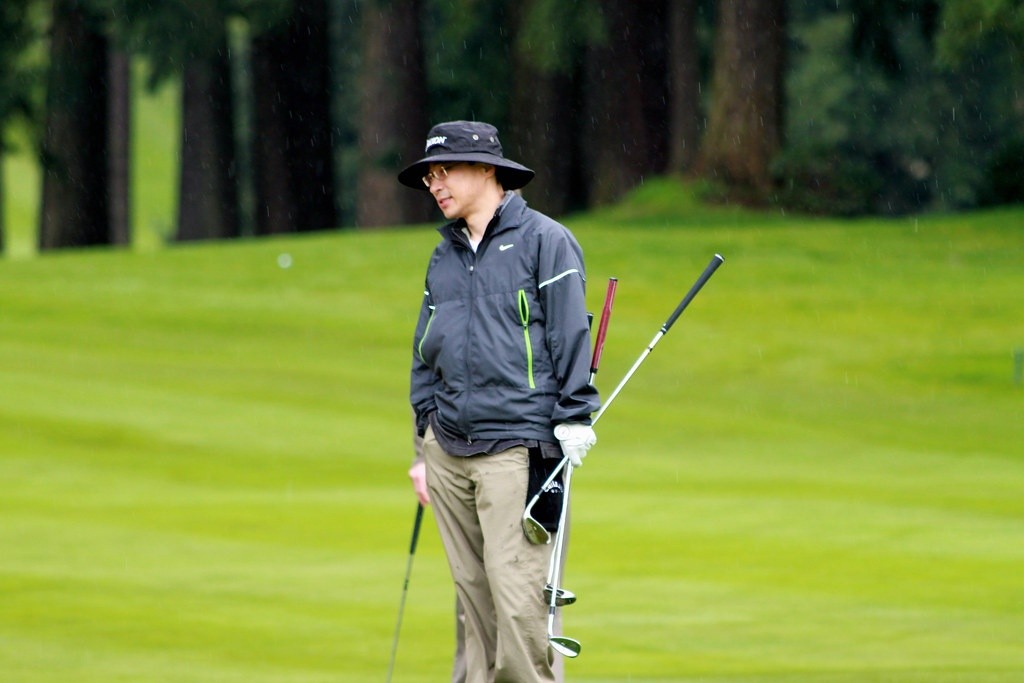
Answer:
[422,161,465,187]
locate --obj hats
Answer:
[397,121,536,192]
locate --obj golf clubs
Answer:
[542,313,597,608]
[383,496,428,683]
[517,251,726,546]
[547,276,619,659]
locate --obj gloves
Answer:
[553,423,596,468]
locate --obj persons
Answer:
[399,120,602,683]
